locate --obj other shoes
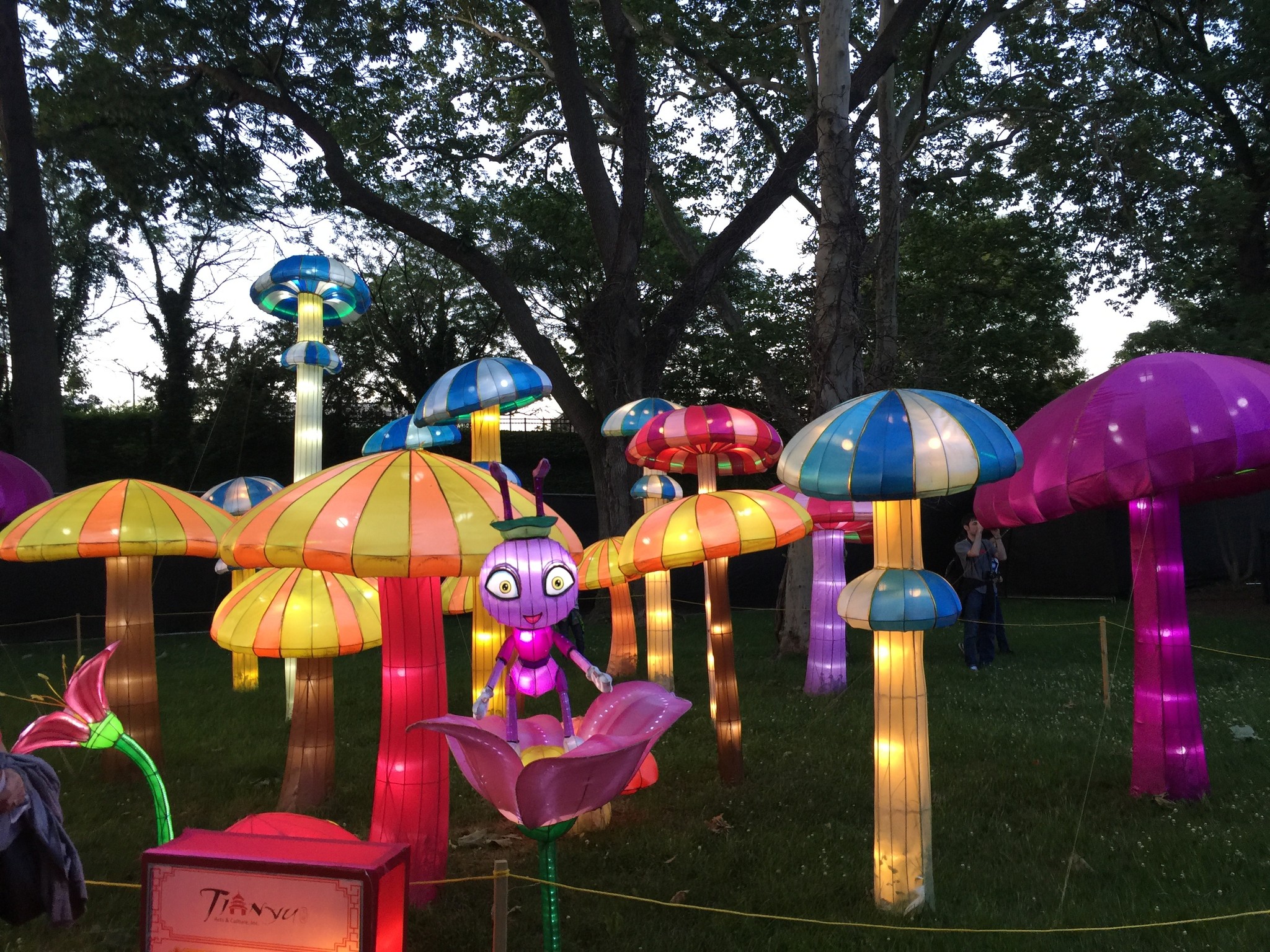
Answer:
[985,663,992,667]
[958,643,965,653]
[970,666,977,670]
[999,648,1013,654]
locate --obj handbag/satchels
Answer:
[0,818,50,924]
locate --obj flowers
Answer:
[403,681,690,951]
[0,641,175,847]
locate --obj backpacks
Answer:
[945,557,967,604]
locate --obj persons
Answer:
[555,598,585,661]
[0,731,25,815]
[954,512,1013,672]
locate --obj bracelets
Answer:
[994,536,1002,540]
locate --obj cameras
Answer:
[982,571,996,580]
